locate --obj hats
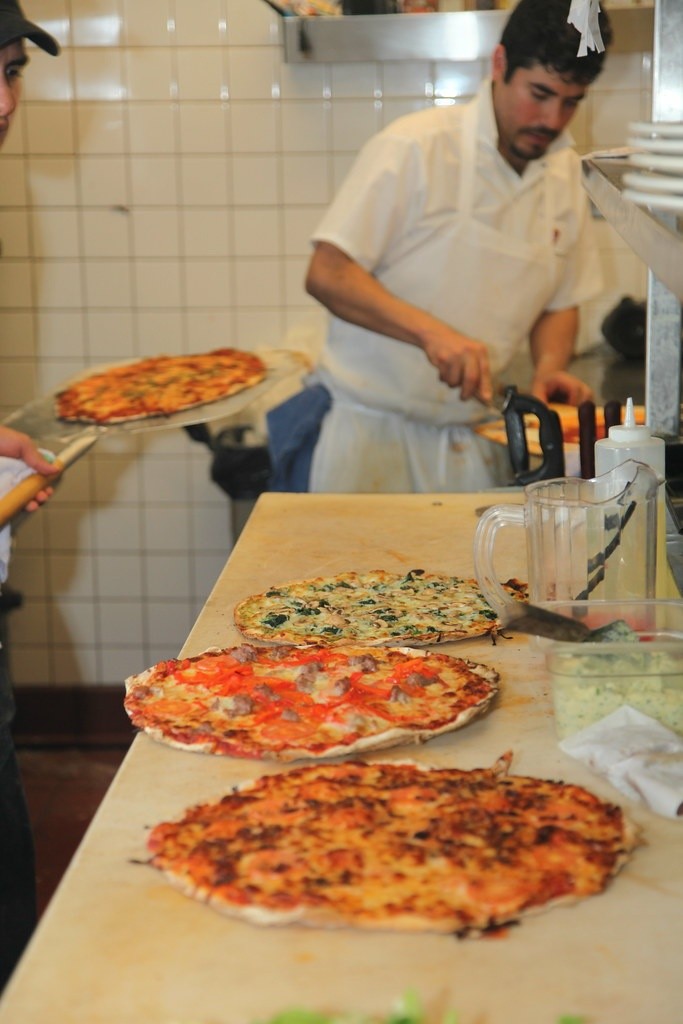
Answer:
[1,0,58,57]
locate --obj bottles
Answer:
[594,393,668,605]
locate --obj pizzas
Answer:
[118,570,641,936]
[474,399,648,458]
[54,347,265,423]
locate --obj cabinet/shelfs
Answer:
[268,0,515,68]
[583,3,683,596]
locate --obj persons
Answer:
[1,0,66,1001]
[304,0,617,491]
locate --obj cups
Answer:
[470,453,655,649]
